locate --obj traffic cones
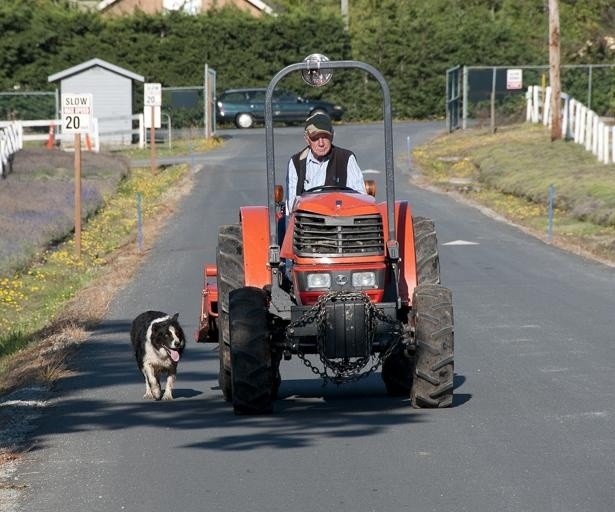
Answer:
[85,133,92,153]
[45,124,55,151]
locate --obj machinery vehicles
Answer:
[193,52,456,418]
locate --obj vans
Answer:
[216,86,344,130]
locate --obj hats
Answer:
[305,114,334,138]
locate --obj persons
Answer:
[282,107,367,302]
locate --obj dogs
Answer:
[130,311,186,401]
